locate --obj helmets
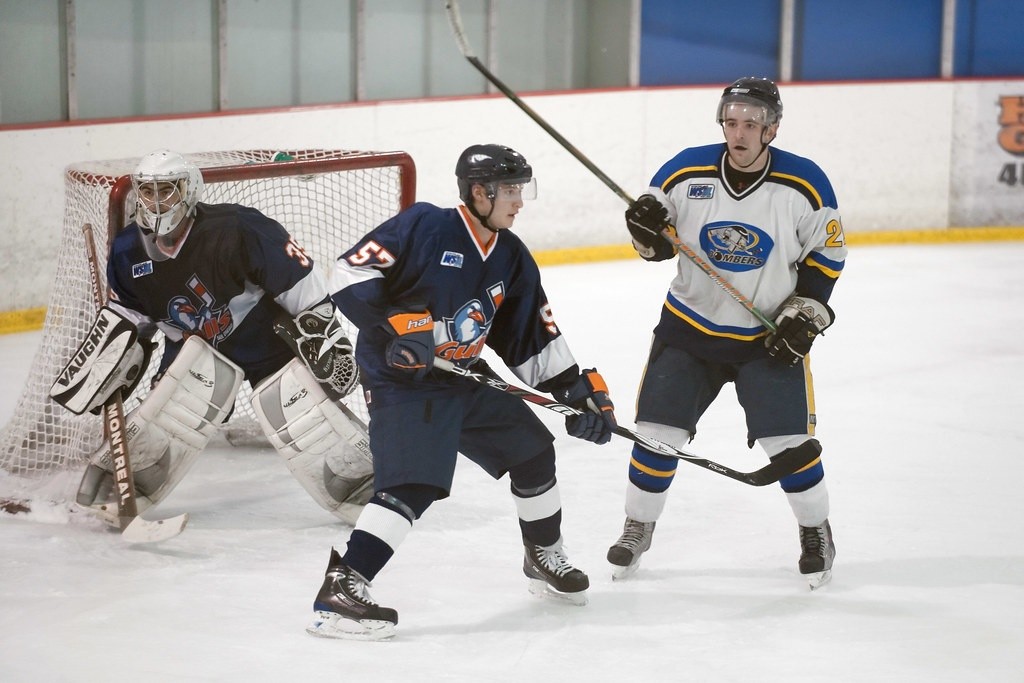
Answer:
[716,76,784,130]
[132,150,204,238]
[455,144,533,203]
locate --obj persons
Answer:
[606,76,848,588]
[304,146,618,639]
[46,149,374,528]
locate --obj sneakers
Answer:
[798,518,836,592]
[521,534,590,608]
[607,515,657,582]
[307,545,399,644]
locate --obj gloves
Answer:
[555,366,617,446]
[373,306,435,384]
[624,199,671,260]
[764,292,835,367]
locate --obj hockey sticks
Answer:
[431,355,825,490]
[81,222,192,546]
[442,1,779,335]
